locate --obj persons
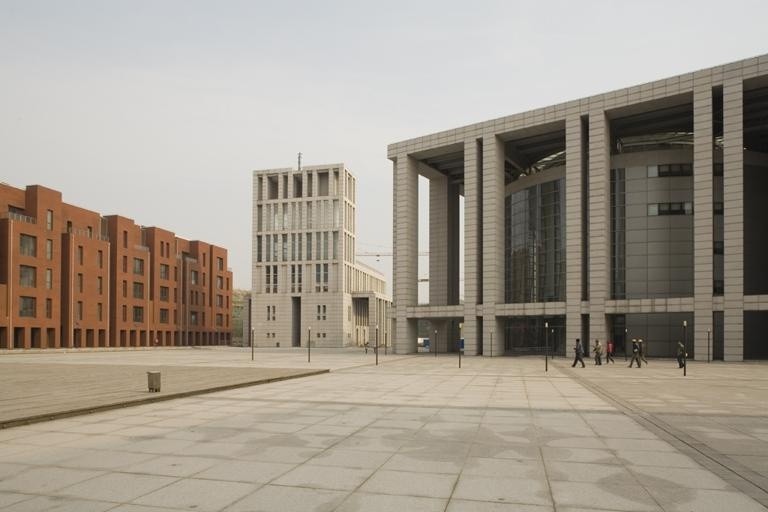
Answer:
[627,340,641,368]
[571,338,586,368]
[605,342,615,365]
[592,340,602,365]
[676,341,688,368]
[638,339,648,366]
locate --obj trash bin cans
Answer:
[147,371,162,393]
[373,345,377,353]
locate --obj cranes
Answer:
[355,250,429,262]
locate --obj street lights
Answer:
[707,327,711,364]
[490,329,493,357]
[308,326,311,363]
[545,322,549,372]
[624,328,628,362]
[251,327,255,360]
[551,328,555,360]
[434,330,438,357]
[458,322,462,368]
[375,324,379,366]
[683,321,687,377]
